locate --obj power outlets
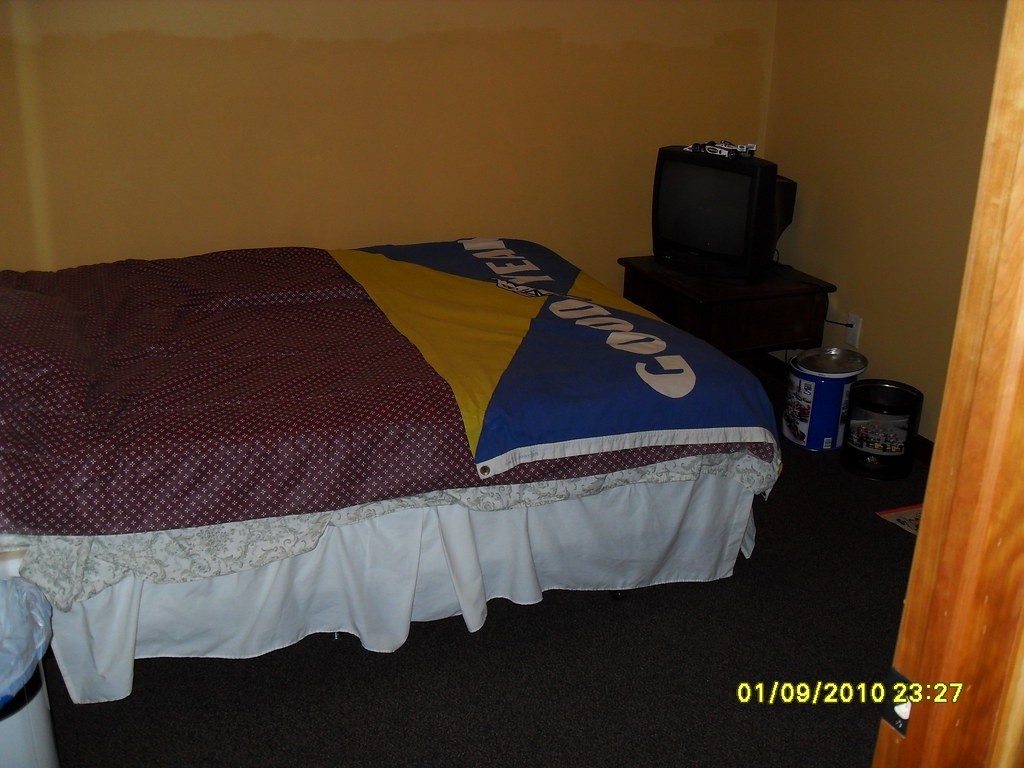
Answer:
[843,313,863,350]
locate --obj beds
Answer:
[0,236,784,707]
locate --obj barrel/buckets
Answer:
[781,345,868,452]
[845,378,924,456]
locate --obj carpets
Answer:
[871,496,923,541]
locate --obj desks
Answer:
[617,255,838,393]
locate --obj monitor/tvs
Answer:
[652,145,797,288]
